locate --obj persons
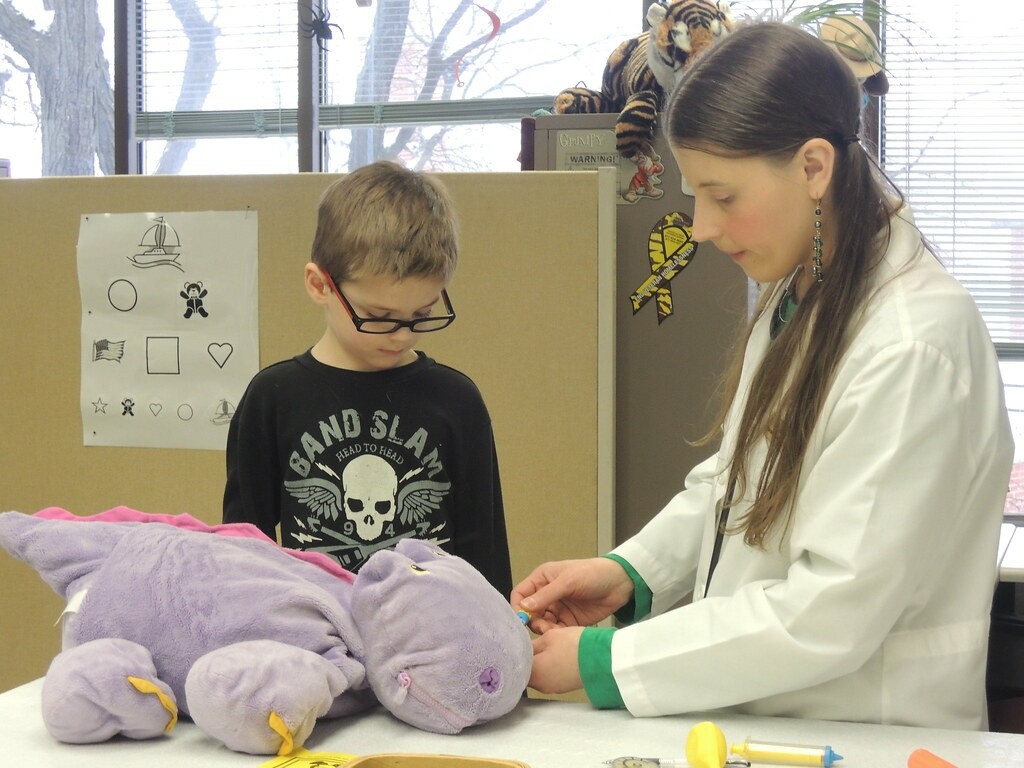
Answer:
[225,163,513,605]
[510,24,1015,732]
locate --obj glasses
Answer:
[317,263,456,334]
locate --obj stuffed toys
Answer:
[554,0,736,159]
[1,509,532,755]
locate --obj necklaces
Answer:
[779,266,812,323]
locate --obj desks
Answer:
[0,673,1024,768]
